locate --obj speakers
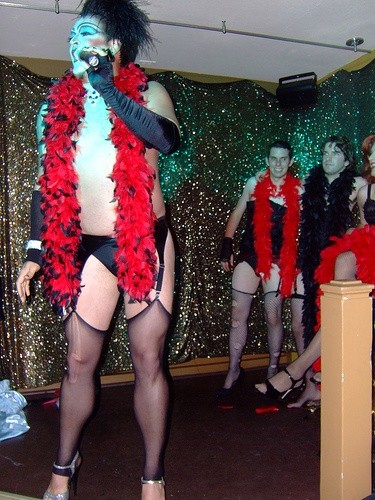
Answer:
[275,78,317,112]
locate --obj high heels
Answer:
[140,475,165,500]
[43,449,83,500]
[215,366,244,398]
[252,365,303,402]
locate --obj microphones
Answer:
[74,46,98,66]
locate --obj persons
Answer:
[219,141,305,400]
[296,137,356,355]
[357,136,375,226]
[256,225,375,410]
[14,0,181,500]
